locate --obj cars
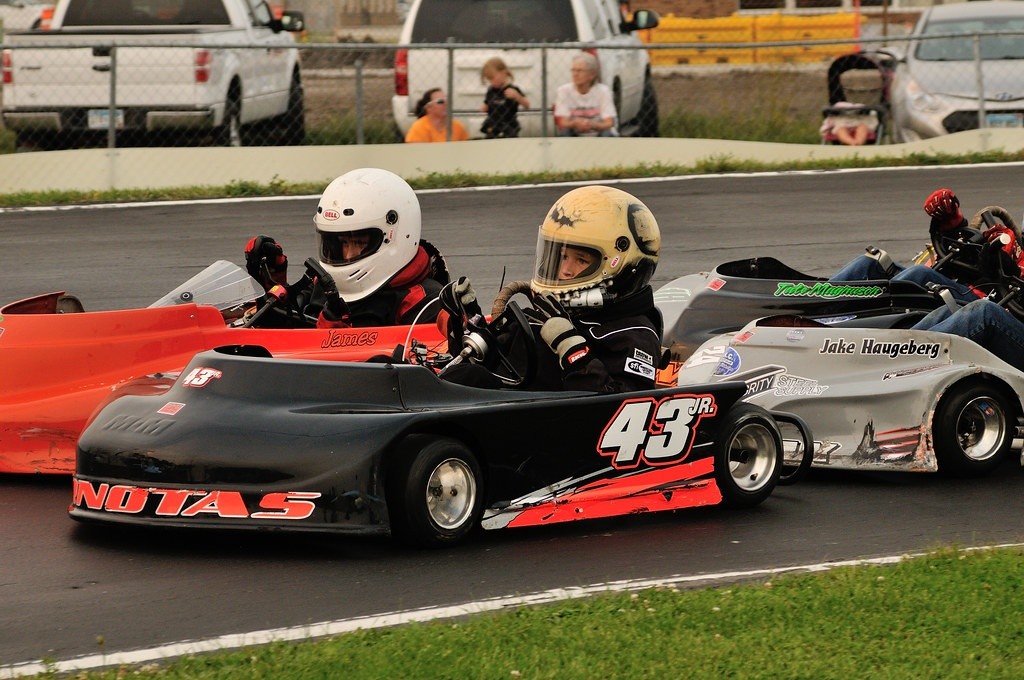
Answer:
[887,0,1024,138]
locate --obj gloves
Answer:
[984,225,1024,276]
[522,292,585,371]
[439,275,488,331]
[317,297,353,330]
[245,236,287,294]
[924,188,964,229]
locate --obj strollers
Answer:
[819,48,898,146]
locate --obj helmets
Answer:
[529,186,662,303]
[313,168,421,302]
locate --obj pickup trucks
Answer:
[0,0,306,149]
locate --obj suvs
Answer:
[392,0,659,142]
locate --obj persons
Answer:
[245,168,450,329]
[366,185,661,391]
[480,58,530,139]
[832,190,1024,372]
[554,52,619,136]
[820,101,876,145]
[404,86,468,142]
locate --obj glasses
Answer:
[423,98,447,109]
